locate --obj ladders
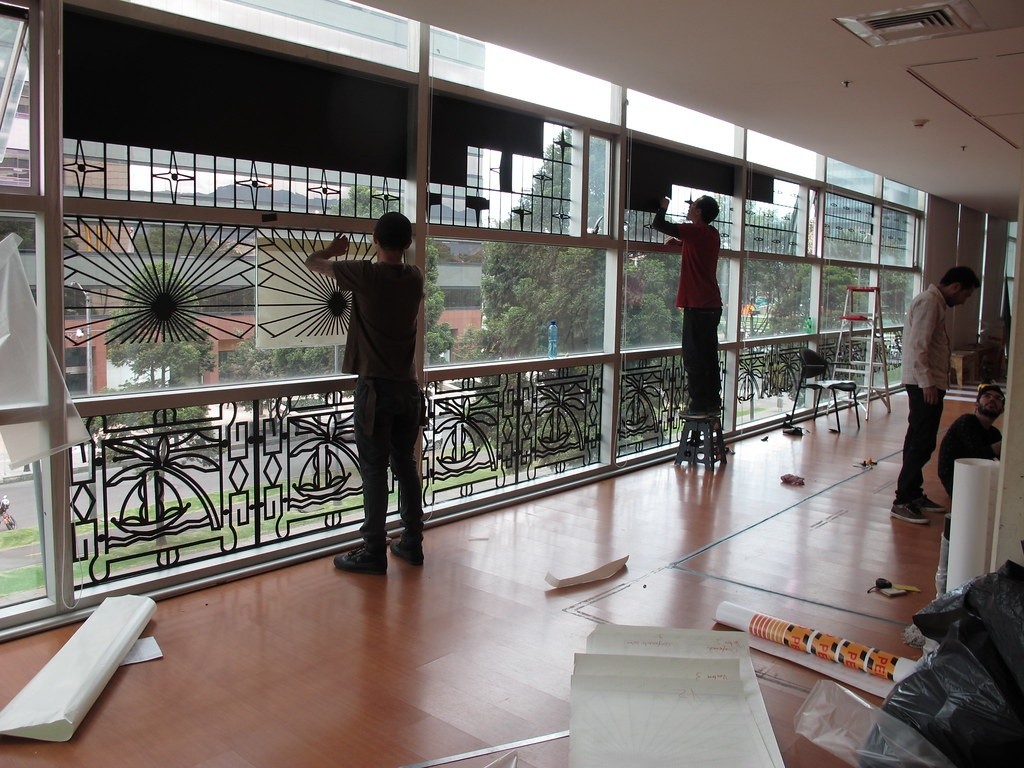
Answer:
[826,285,892,420]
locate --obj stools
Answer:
[675,417,727,470]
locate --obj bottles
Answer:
[547,320,559,359]
[806,317,814,335]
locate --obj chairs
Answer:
[789,347,862,434]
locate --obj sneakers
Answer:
[332,545,387,575]
[709,409,721,418]
[678,409,709,419]
[890,502,931,524]
[916,494,946,512]
[390,540,424,566]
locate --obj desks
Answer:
[949,350,977,386]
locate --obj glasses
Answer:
[982,393,1004,401]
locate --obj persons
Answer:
[889,265,982,524]
[303,210,428,576]
[937,383,1006,513]
[651,195,724,420]
[1,495,9,516]
